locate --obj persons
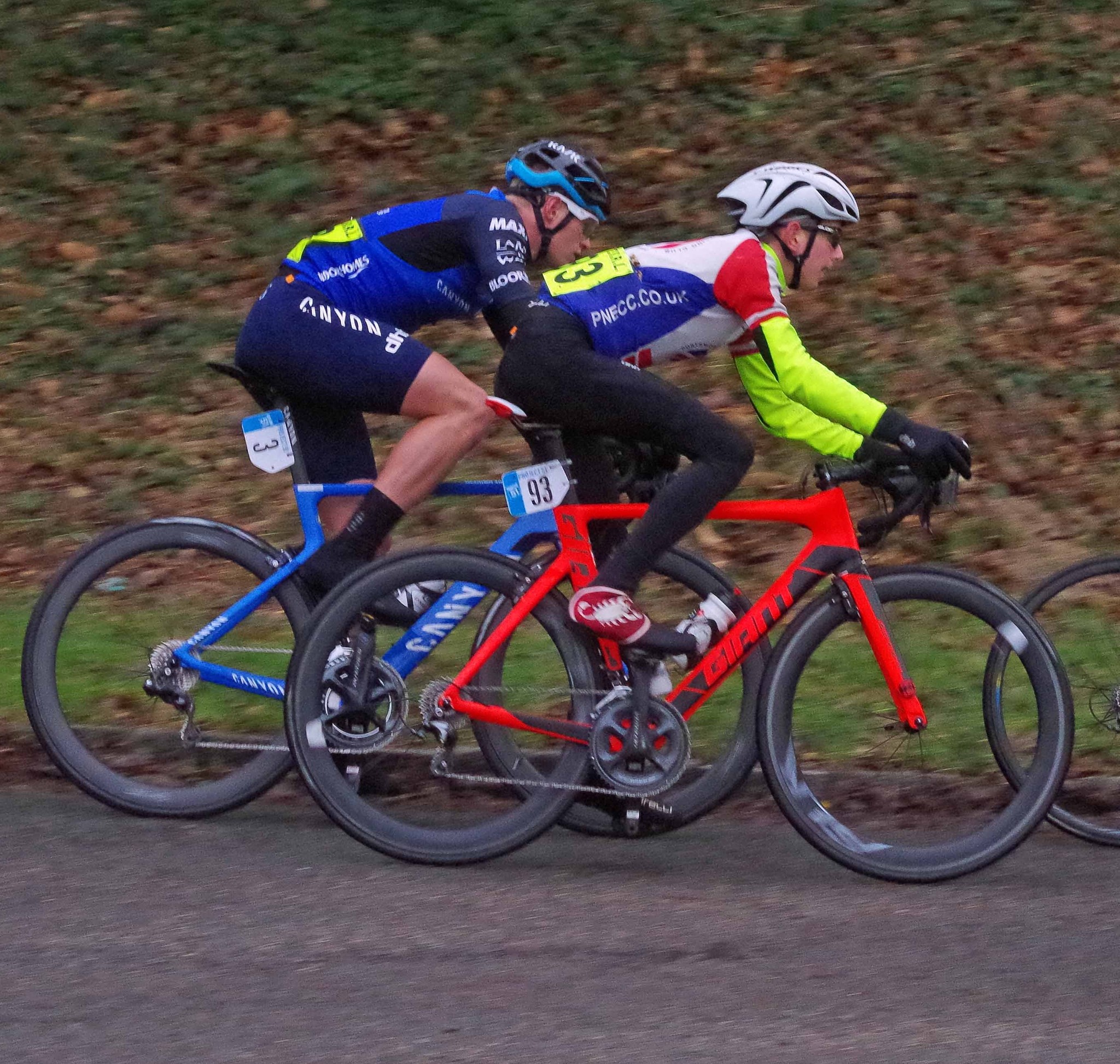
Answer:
[492,161,973,655]
[233,139,611,794]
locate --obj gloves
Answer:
[856,438,901,467]
[899,420,974,482]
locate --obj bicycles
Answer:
[18,359,774,842]
[283,392,1120,887]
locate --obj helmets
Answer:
[505,140,611,221]
[717,161,860,228]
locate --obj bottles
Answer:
[394,572,447,617]
[661,585,744,669]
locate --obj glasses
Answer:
[801,225,842,246]
[582,220,598,238]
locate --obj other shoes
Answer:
[335,751,402,794]
[303,546,420,630]
[576,770,631,819]
[567,573,697,655]
